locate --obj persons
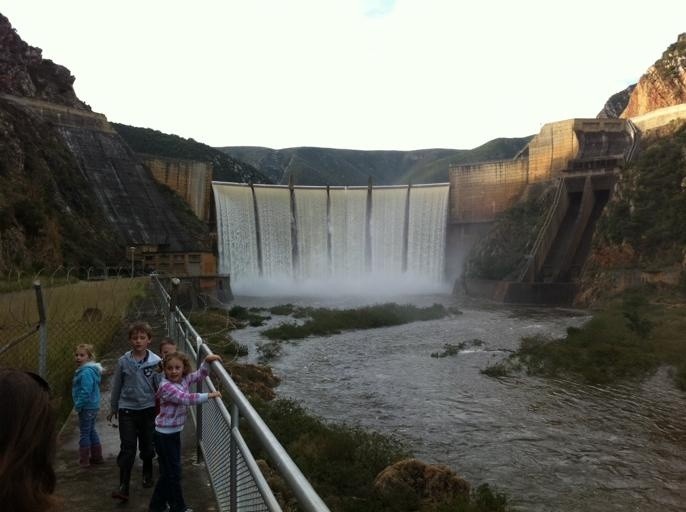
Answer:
[70,343,107,468]
[151,337,177,450]
[0,368,67,510]
[146,350,225,511]
[106,320,164,500]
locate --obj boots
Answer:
[89,443,106,465]
[141,457,155,489]
[111,464,132,501]
[75,446,91,468]
